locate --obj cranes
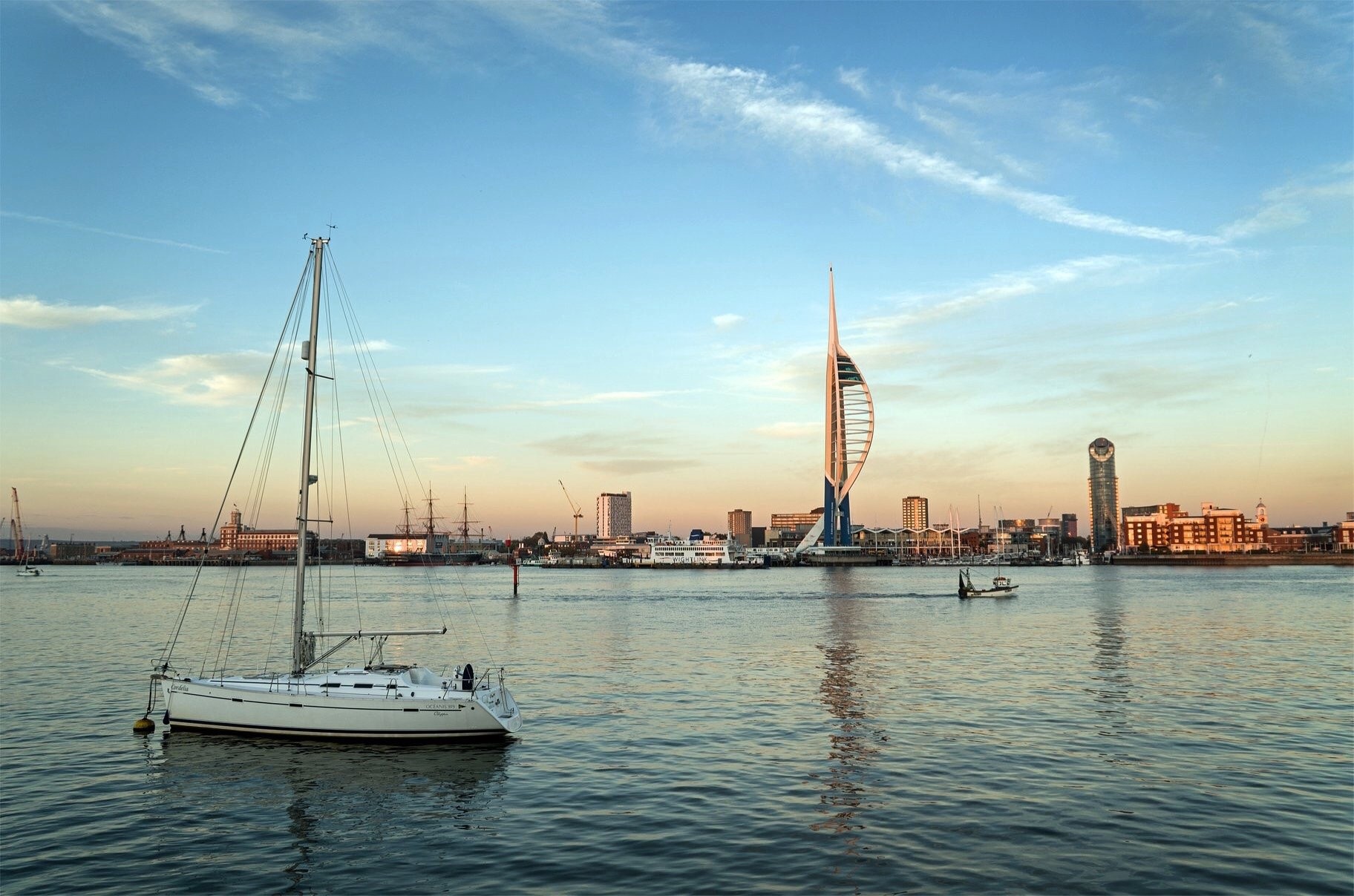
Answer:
[558,479,584,541]
[9,486,37,560]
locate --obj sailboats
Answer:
[16,535,44,576]
[152,226,523,743]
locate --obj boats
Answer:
[957,506,1019,598]
